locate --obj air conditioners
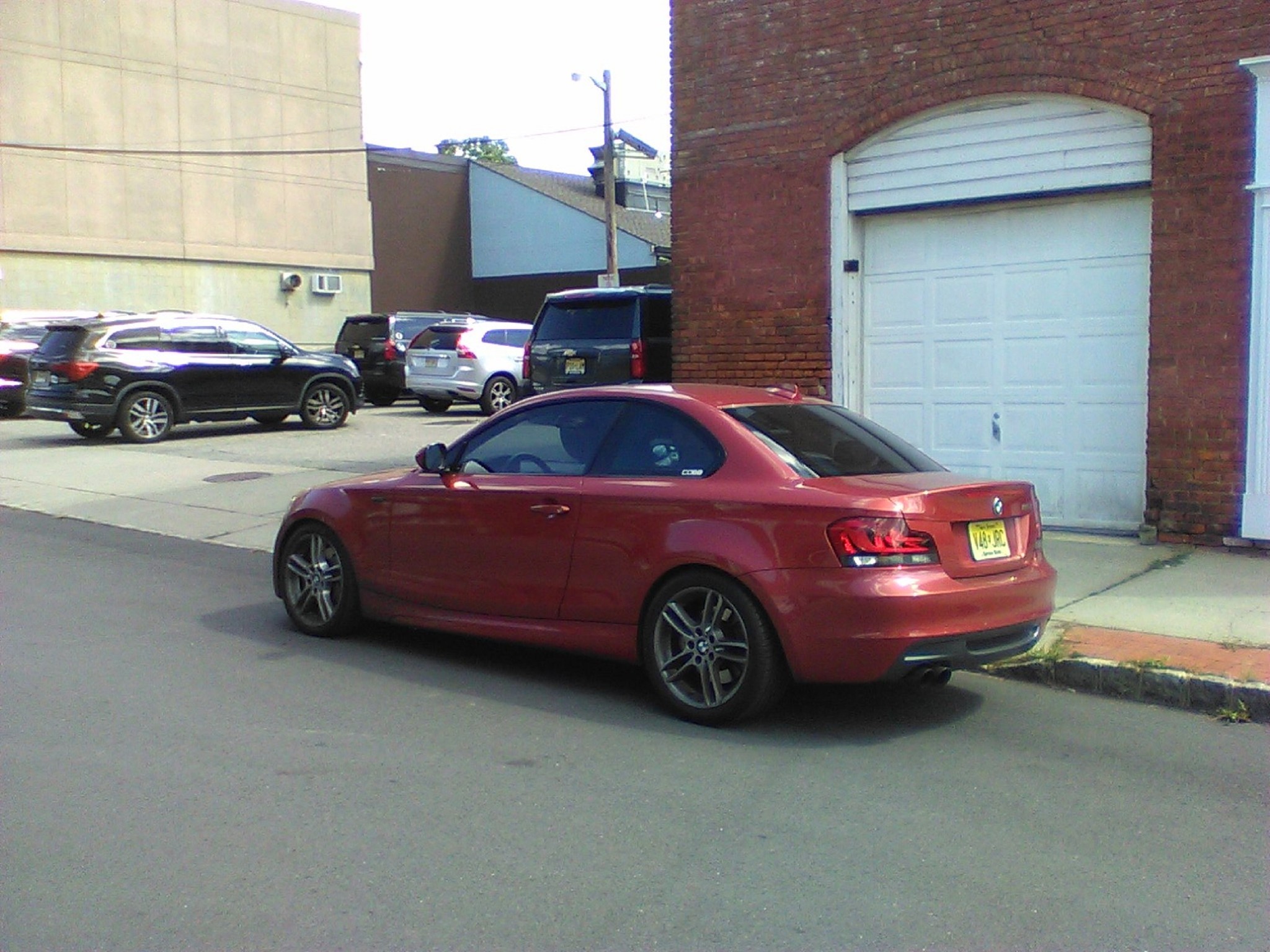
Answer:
[312,273,343,296]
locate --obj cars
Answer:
[0,309,153,421]
[403,318,534,416]
[270,382,1056,728]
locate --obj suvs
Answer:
[335,312,482,404]
[24,307,366,440]
[519,285,677,400]
